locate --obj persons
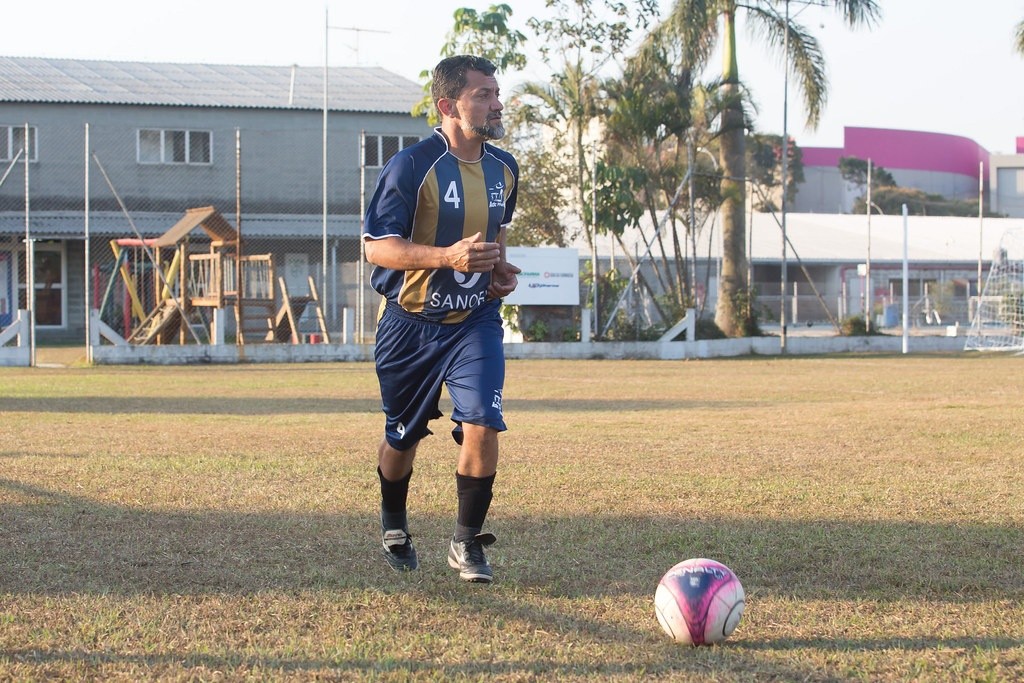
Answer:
[361,55,521,583]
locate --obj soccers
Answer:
[653,558,746,646]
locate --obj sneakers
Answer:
[448,532,497,583]
[380,501,419,574]
